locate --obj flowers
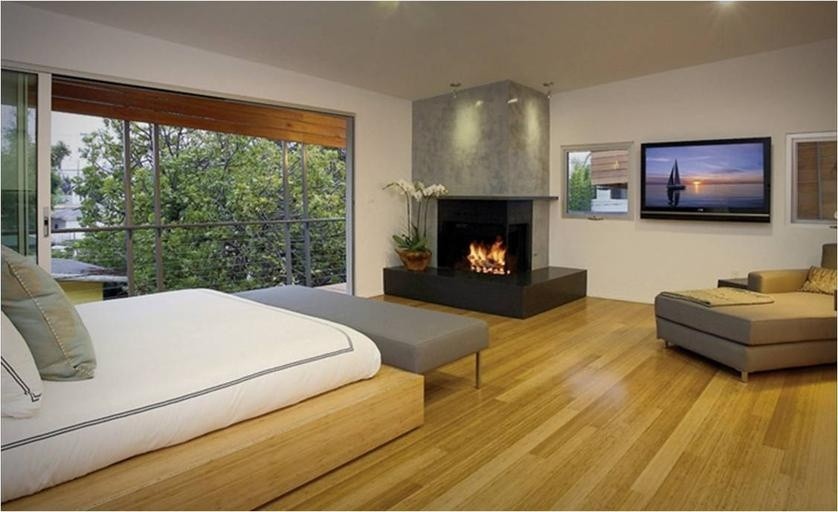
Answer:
[383,177,448,250]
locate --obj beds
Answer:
[2,287,425,511]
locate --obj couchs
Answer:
[232,285,489,389]
[655,243,836,382]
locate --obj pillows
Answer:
[2,311,48,420]
[1,245,96,381]
[800,266,837,295]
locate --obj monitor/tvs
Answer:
[638,136,771,224]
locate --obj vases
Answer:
[395,248,430,271]
